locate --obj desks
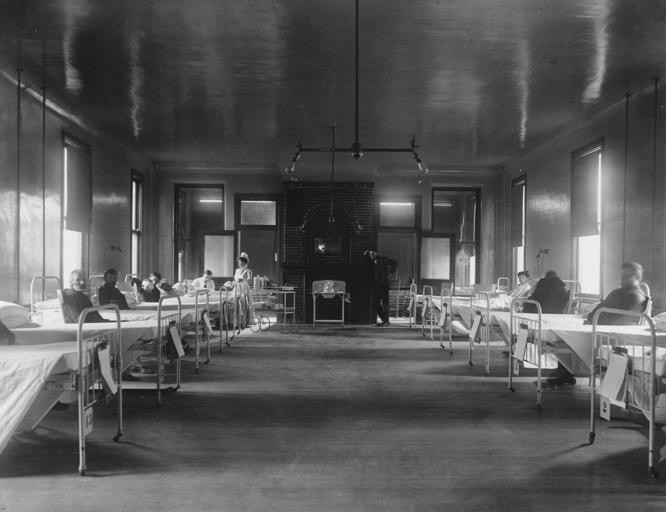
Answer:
[308,279,351,331]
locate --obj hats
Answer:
[237,252,249,262]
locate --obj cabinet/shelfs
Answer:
[249,290,297,318]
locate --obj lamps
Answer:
[349,0,365,161]
[284,133,432,176]
[328,128,337,224]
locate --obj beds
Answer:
[585,306,664,476]
[27,278,213,373]
[1,294,189,406]
[507,296,654,408]
[436,280,583,358]
[124,278,248,334]
[408,277,512,330]
[466,294,603,376]
[87,278,234,352]
[0,301,125,477]
[421,281,536,342]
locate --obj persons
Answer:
[131,271,161,302]
[522,270,570,314]
[192,269,215,292]
[361,248,398,326]
[546,260,646,384]
[507,270,531,299]
[62,269,110,322]
[225,252,255,324]
[98,268,132,310]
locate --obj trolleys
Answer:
[250,277,300,334]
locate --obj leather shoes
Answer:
[379,322,390,327]
